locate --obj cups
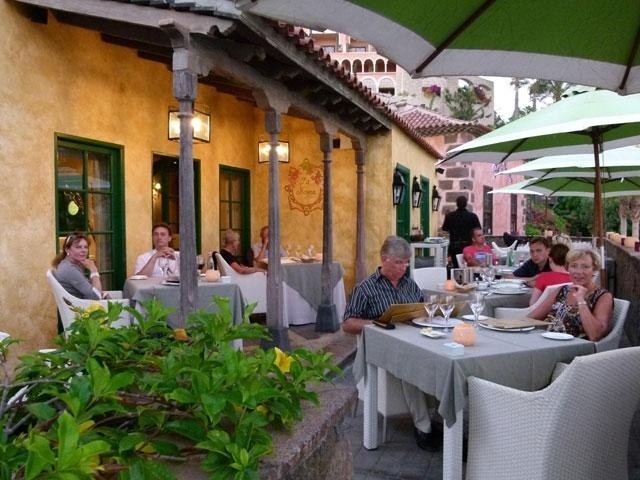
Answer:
[295,246,302,261]
[158,256,169,283]
[485,269,495,292]
[307,245,314,258]
[468,293,486,330]
[423,294,439,331]
[196,256,205,279]
[494,266,504,286]
[479,267,489,287]
[439,296,455,335]
[285,243,293,258]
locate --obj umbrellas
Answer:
[433,85,640,288]
[487,179,551,234]
[236,0,640,97]
[494,178,640,243]
[494,146,640,243]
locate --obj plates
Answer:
[462,314,490,320]
[541,332,574,340]
[479,321,536,332]
[491,288,529,295]
[412,315,463,328]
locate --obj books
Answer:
[381,300,466,325]
[481,318,557,329]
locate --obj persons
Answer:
[461,230,499,279]
[443,196,482,269]
[134,223,183,279]
[219,229,268,276]
[523,243,614,341]
[54,237,111,300]
[252,225,286,267]
[530,245,573,305]
[341,236,443,452]
[509,238,552,280]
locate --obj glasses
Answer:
[567,261,593,272]
[395,260,411,269]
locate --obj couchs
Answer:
[588,299,632,351]
[352,353,409,441]
[464,345,640,479]
[214,252,270,317]
[490,281,575,325]
[414,266,448,296]
[452,253,466,270]
[45,268,132,345]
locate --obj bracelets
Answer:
[578,301,587,304]
[89,272,100,278]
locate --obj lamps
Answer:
[432,186,442,211]
[392,170,407,206]
[412,175,425,210]
[256,138,292,164]
[166,106,212,145]
